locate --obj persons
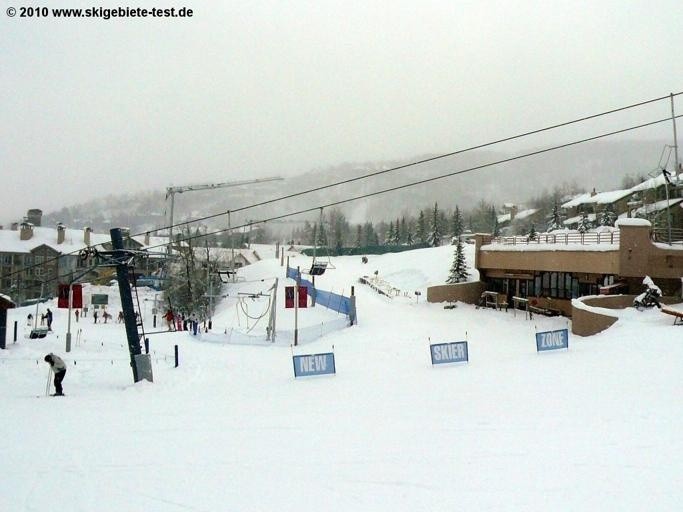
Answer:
[27,314,33,325]
[94,312,97,323]
[41,309,52,331]
[75,310,79,322]
[45,355,66,396]
[119,312,124,323]
[104,312,107,323]
[162,310,196,331]
